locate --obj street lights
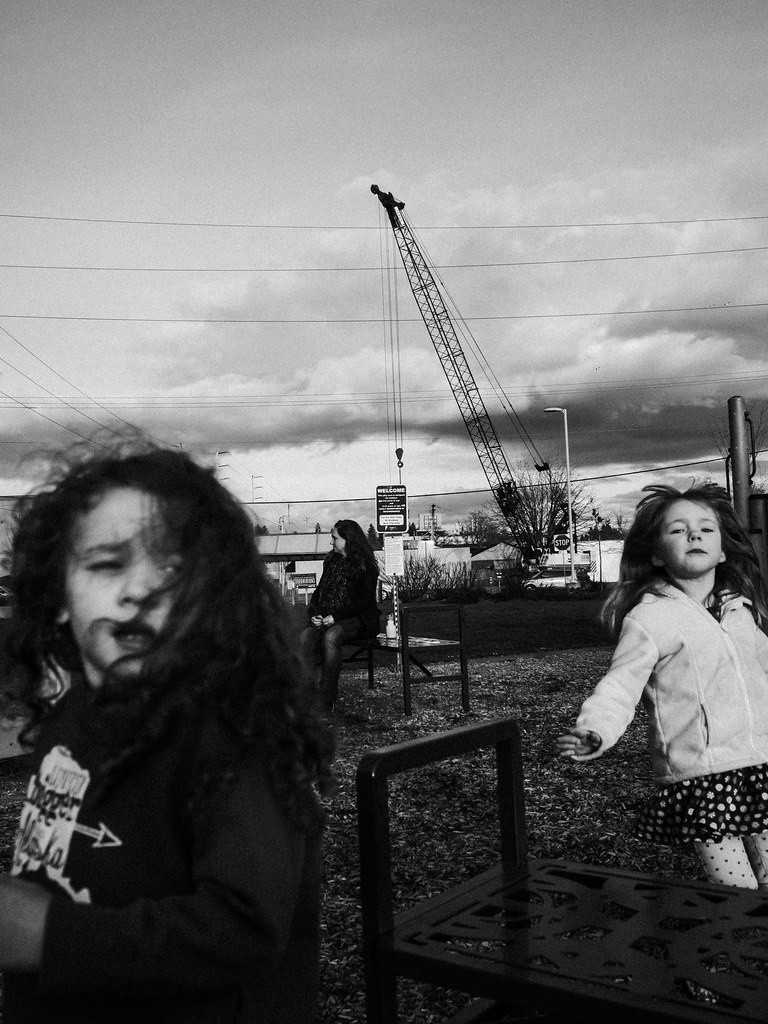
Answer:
[544,407,577,583]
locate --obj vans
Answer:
[523,568,587,591]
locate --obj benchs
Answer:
[356,714,768,1024]
[338,603,470,717]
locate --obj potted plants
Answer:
[385,612,396,639]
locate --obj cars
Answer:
[380,579,394,600]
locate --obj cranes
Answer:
[370,183,574,559]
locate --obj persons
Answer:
[0,452,337,1023]
[305,520,381,713]
[554,479,768,891]
